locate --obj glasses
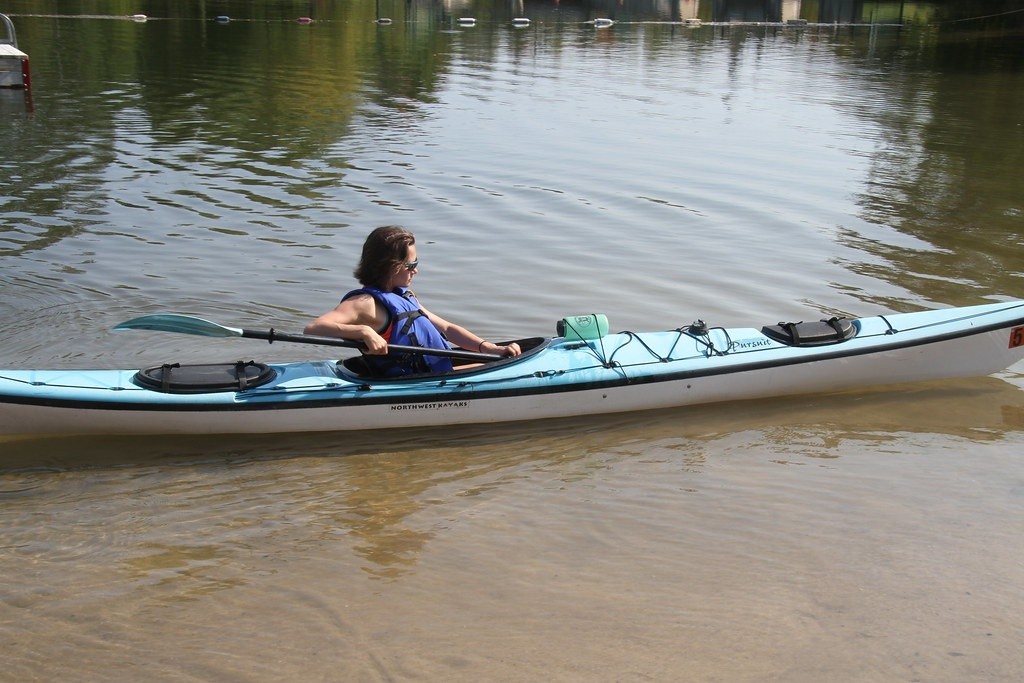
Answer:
[399,257,419,271]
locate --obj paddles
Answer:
[112,314,511,363]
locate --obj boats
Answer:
[1,302,1024,434]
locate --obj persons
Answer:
[304,225,521,375]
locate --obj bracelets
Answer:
[479,341,486,353]
